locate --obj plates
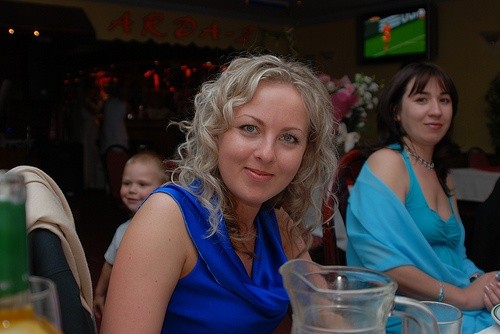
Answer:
[491,304,500,326]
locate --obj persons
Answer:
[383,23,391,49]
[93,152,169,319]
[99,55,355,334]
[71,80,132,196]
[343,65,500,334]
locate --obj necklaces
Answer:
[406,146,434,169]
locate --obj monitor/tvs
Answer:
[355,0,439,65]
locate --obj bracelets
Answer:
[437,282,445,302]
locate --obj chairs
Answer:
[320,148,362,270]
[465,145,488,167]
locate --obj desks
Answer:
[446,166,500,273]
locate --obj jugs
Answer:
[279,258,439,334]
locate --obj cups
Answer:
[402,301,463,334]
[0,275,63,334]
